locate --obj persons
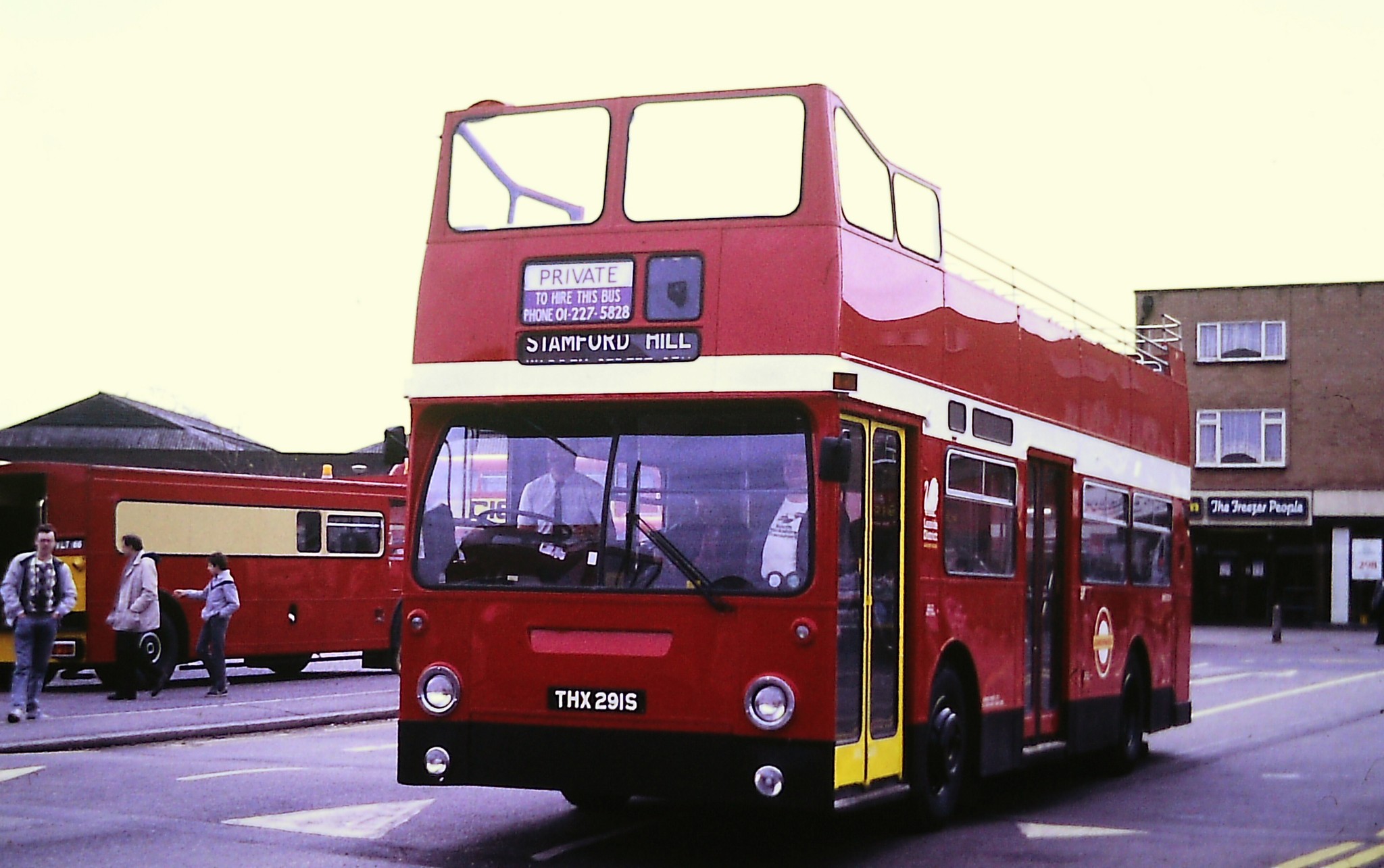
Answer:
[106,536,167,700]
[515,438,617,543]
[1372,578,1384,645]
[637,493,748,576]
[744,450,857,595]
[0,523,77,723]
[172,552,240,696]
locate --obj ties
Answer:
[552,481,564,524]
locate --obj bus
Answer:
[392,76,1200,856]
[0,449,404,695]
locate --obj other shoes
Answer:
[8,710,22,722]
[208,686,229,695]
[107,692,137,700]
[151,672,168,697]
[224,678,230,684]
[25,702,40,719]
